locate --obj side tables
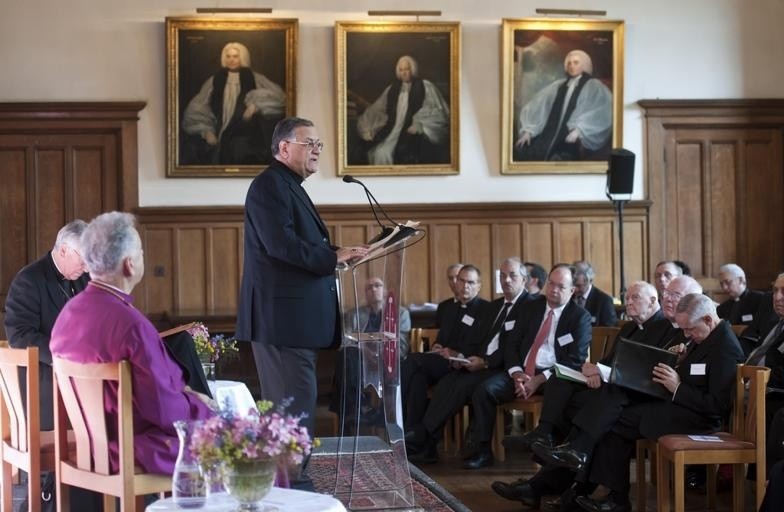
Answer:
[144,486,346,512]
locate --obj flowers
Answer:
[192,396,322,477]
[187,323,239,365]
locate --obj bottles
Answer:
[170,418,216,510]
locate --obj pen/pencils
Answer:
[685,339,693,346]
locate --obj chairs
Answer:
[53,358,172,512]
[0,346,78,512]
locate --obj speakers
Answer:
[607,148,635,193]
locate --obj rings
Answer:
[351,248,358,253]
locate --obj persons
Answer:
[694,273,784,495]
[48,211,291,493]
[405,256,540,463]
[433,263,465,329]
[351,265,491,430]
[350,54,450,164]
[3,218,213,430]
[739,280,780,360]
[524,262,548,299]
[456,263,592,472]
[500,280,663,466]
[232,116,369,490]
[654,261,683,292]
[514,49,613,161]
[571,261,617,327]
[490,275,704,508]
[532,293,745,511]
[182,42,287,167]
[716,263,765,337]
[328,277,411,417]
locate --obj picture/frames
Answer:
[164,13,300,179]
[332,19,463,178]
[499,16,626,176]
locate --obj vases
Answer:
[171,419,208,510]
[219,458,283,512]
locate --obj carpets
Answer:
[270,448,461,511]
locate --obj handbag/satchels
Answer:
[20,472,100,511]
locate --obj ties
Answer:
[576,296,586,309]
[484,302,514,360]
[61,279,75,302]
[524,309,556,378]
[742,318,784,385]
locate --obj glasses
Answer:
[282,139,325,150]
[662,290,683,301]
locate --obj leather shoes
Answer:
[399,431,438,452]
[462,444,496,469]
[501,428,555,452]
[531,441,588,484]
[576,494,634,512]
[491,479,545,508]
[545,490,580,510]
[406,449,440,464]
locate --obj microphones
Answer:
[343,175,415,248]
[344,175,394,244]
